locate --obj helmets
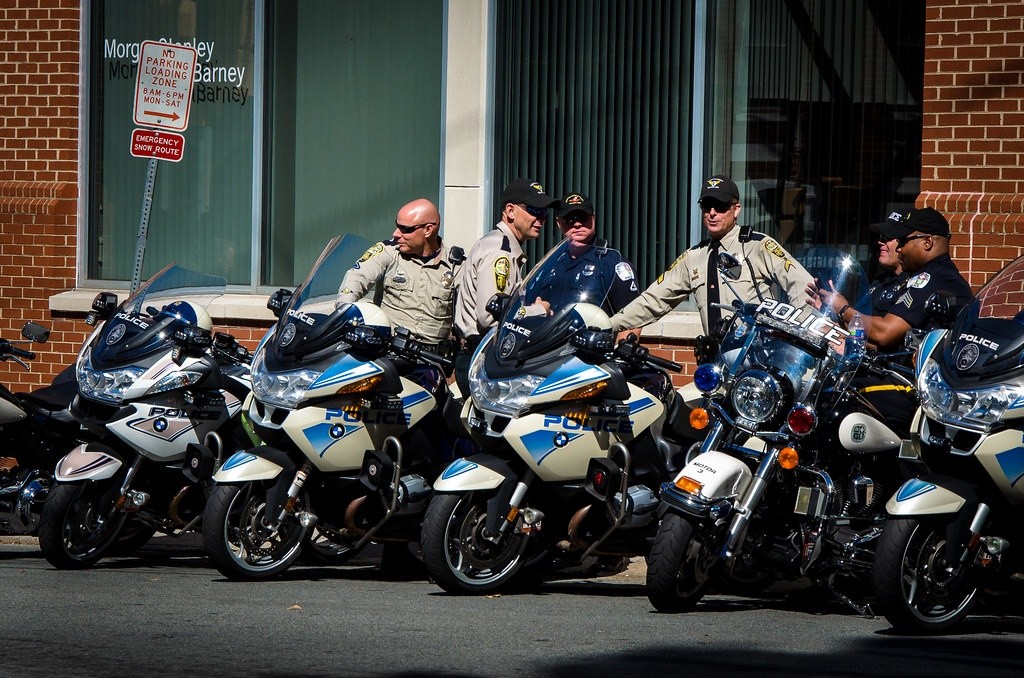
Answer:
[562,300,612,336]
[340,301,392,362]
[152,300,215,334]
[719,310,750,369]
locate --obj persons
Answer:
[453,180,563,399]
[609,175,815,336]
[805,206,978,442]
[525,193,641,345]
[337,200,466,354]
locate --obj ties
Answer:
[707,239,722,336]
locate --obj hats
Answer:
[501,179,562,210]
[870,208,917,238]
[559,192,594,219]
[883,206,952,238]
[698,174,740,204]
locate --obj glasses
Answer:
[560,213,592,228]
[698,199,738,214]
[896,233,933,248]
[879,233,900,243]
[395,218,436,234]
[512,202,550,220]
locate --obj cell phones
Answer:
[818,270,830,295]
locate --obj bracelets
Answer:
[838,305,850,321]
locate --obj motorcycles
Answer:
[420,234,710,596]
[0,321,89,536]
[873,255,1023,634]
[38,265,267,569]
[200,231,468,583]
[646,247,919,619]
